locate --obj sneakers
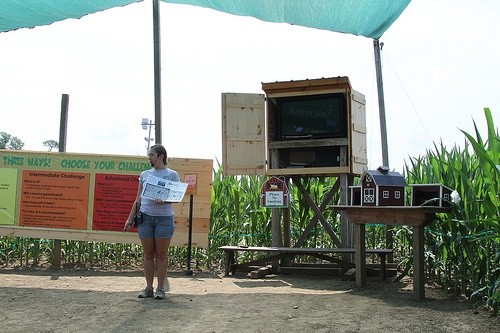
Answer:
[138,287,154,298]
[154,288,165,299]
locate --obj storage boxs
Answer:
[409,184,455,207]
[220,77,368,177]
[359,165,407,205]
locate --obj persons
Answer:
[123,144,180,299]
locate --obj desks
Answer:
[326,205,454,303]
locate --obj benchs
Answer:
[218,245,393,283]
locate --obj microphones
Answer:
[151,154,160,166]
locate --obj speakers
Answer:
[277,93,346,141]
